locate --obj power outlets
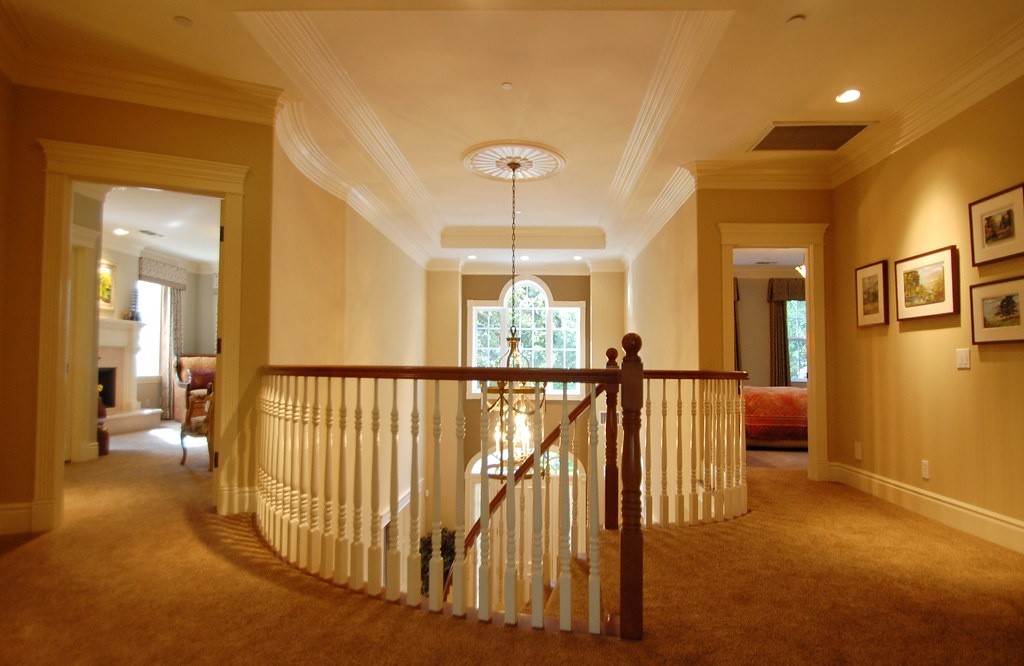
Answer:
[956,348,971,369]
[922,460,930,479]
[854,441,863,461]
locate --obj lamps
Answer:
[462,141,567,389]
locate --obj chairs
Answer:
[173,390,217,471]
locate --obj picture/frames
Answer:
[895,245,960,322]
[98,263,116,310]
[969,275,1024,346]
[968,182,1024,267]
[855,259,890,328]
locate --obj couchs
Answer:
[173,354,215,424]
[738,385,808,449]
